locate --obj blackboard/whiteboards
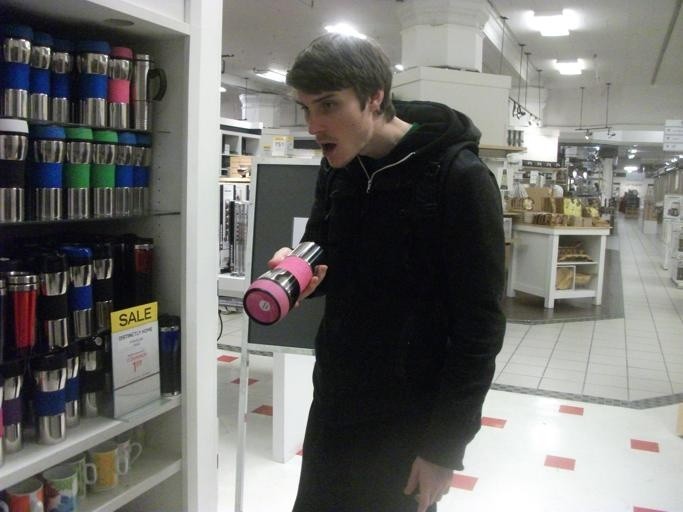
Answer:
[242,158,325,350]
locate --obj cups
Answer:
[242,239,329,330]
[105,435,143,480]
[85,440,129,493]
[4,477,45,512]
[63,452,97,500]
[0,11,179,468]
[42,466,79,512]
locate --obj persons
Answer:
[263,30,510,511]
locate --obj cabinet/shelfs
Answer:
[220,132,261,183]
[0,0,188,512]
[505,223,614,307]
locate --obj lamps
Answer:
[584,129,593,140]
[511,101,526,119]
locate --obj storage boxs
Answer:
[224,155,256,179]
[660,192,682,290]
